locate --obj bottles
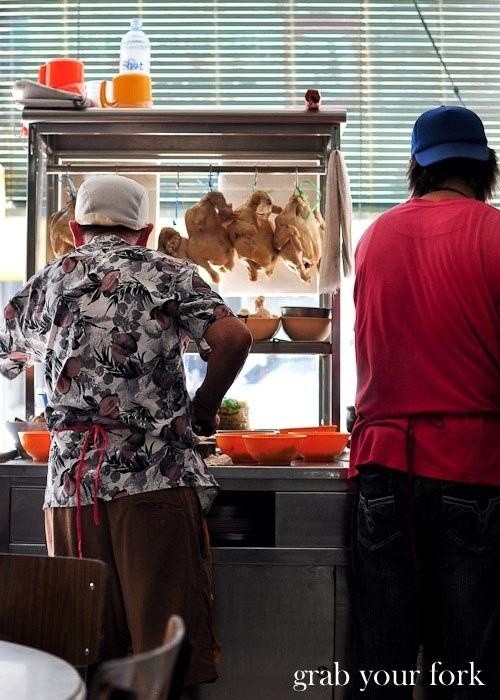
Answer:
[119,18,153,78]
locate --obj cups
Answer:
[100,72,154,110]
[40,57,85,97]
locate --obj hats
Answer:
[411,105,489,167]
[74,175,149,230]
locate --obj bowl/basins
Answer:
[279,306,331,341]
[215,423,352,466]
[18,430,52,465]
[236,317,281,343]
[6,420,47,463]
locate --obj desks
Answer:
[0,641,89,695]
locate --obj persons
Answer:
[1,176,254,699]
[346,106,498,696]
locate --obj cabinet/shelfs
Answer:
[19,107,342,456]
[0,444,499,695]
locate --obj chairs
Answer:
[0,553,110,684]
[92,615,187,694]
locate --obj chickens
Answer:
[272,195,325,285]
[49,197,77,260]
[225,190,283,282]
[184,192,240,283]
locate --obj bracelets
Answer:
[195,389,219,416]
